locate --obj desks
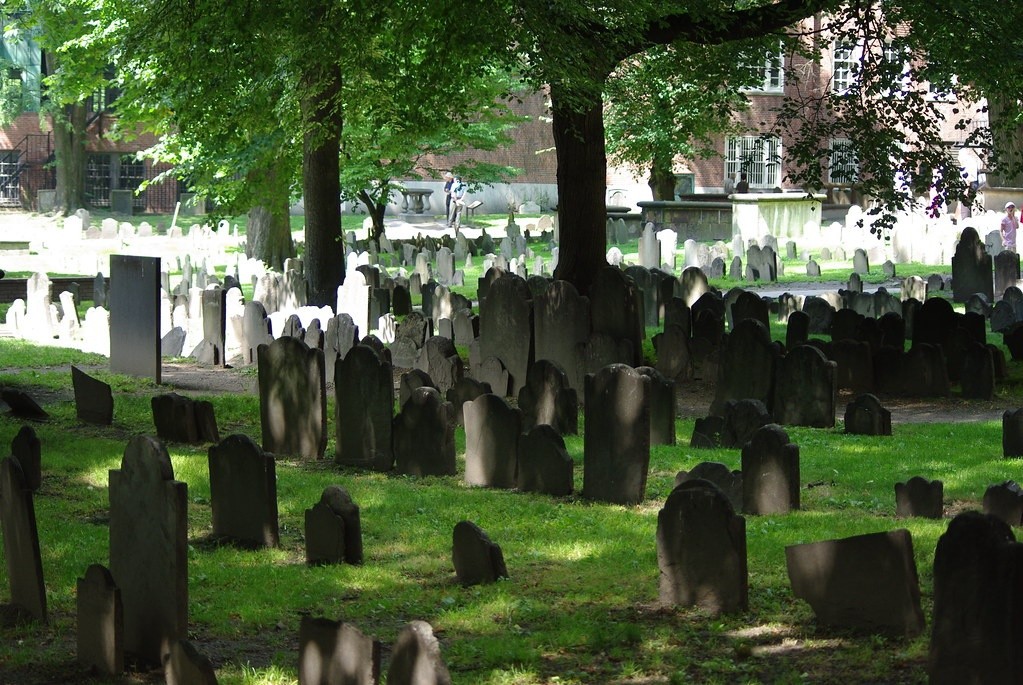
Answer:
[799,182,853,203]
[401,187,433,215]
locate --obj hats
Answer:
[1006,202,1016,209]
[446,172,454,179]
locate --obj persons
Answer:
[443,171,466,228]
[999,202,1019,253]
